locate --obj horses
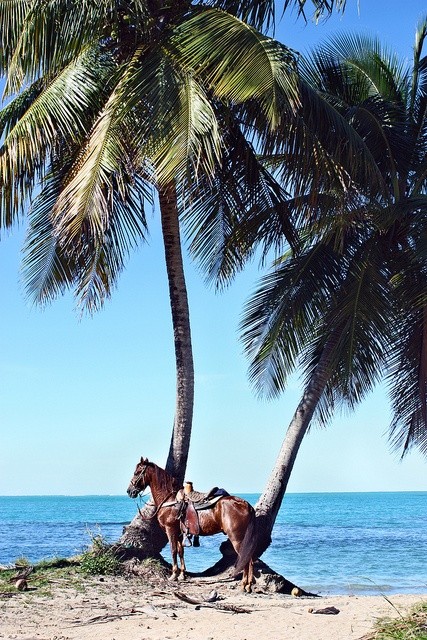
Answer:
[127,456,259,594]
